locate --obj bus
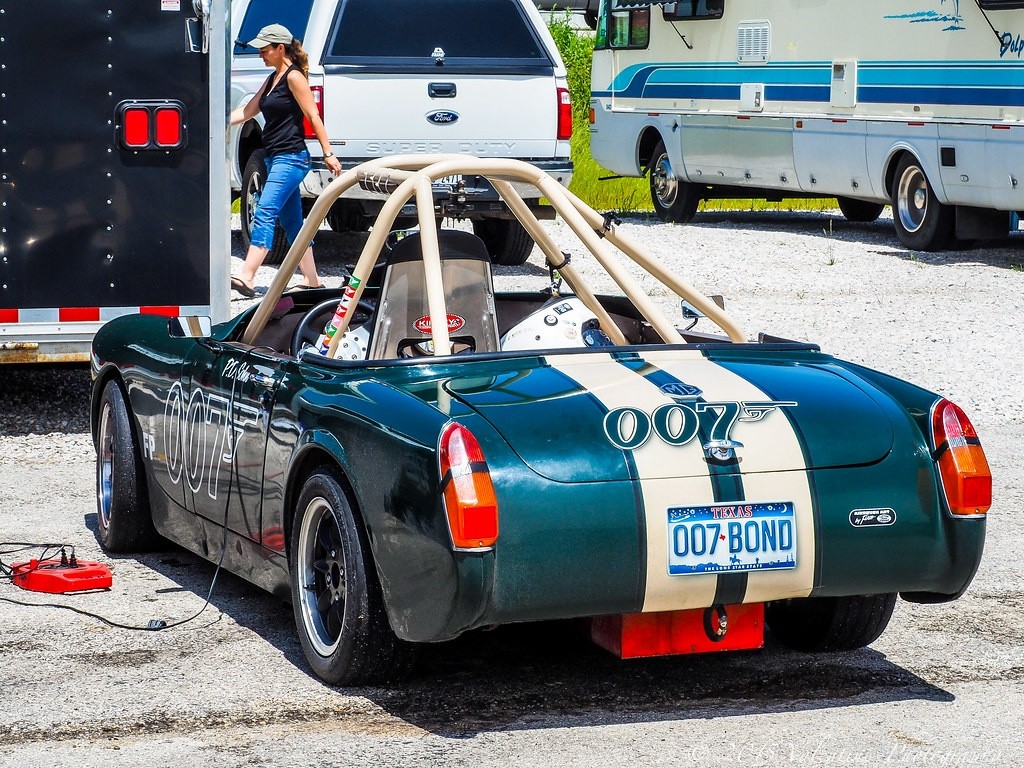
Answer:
[590,0,1024,251]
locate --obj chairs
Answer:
[364,229,503,360]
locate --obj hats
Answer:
[246,22,295,50]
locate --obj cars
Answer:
[90,152,992,686]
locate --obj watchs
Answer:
[323,152,333,160]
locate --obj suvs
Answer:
[225,0,575,266]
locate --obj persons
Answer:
[230,24,342,298]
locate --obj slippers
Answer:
[287,284,326,292]
[230,277,255,297]
